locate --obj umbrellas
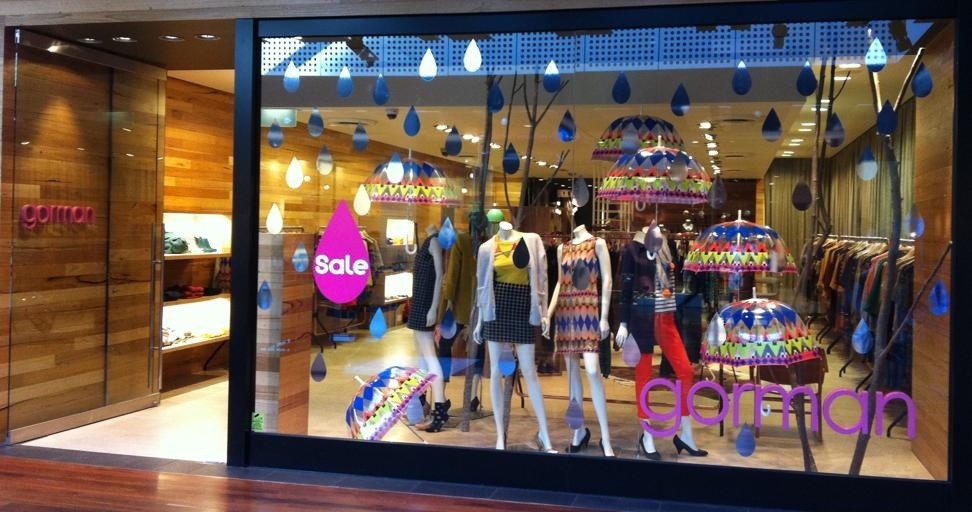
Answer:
[596,132,713,261]
[699,284,817,433]
[680,208,801,301]
[589,104,685,213]
[343,365,438,444]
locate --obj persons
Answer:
[407,222,453,435]
[470,218,560,457]
[615,226,708,462]
[431,210,492,417]
[547,220,616,460]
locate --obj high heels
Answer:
[471,397,480,412]
[599,438,615,458]
[673,434,708,456]
[639,433,661,459]
[534,432,558,453]
[565,428,591,453]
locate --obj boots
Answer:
[414,399,451,432]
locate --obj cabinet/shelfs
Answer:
[159,212,232,391]
